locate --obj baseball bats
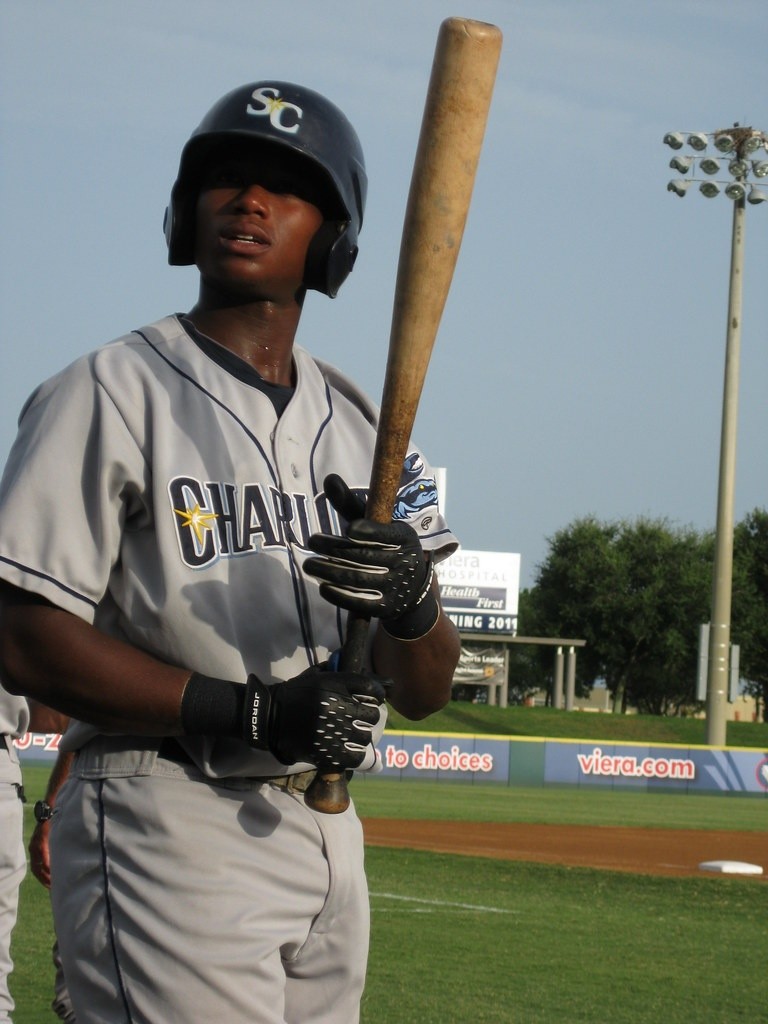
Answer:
[304,16,504,814]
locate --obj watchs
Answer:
[34,800,53,822]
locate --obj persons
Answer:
[476,674,554,707]
[1,81,460,1023]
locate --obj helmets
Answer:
[163,80,369,299]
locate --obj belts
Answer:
[0,734,7,749]
[95,734,354,792]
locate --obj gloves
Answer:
[180,660,386,773]
[302,475,440,641]
